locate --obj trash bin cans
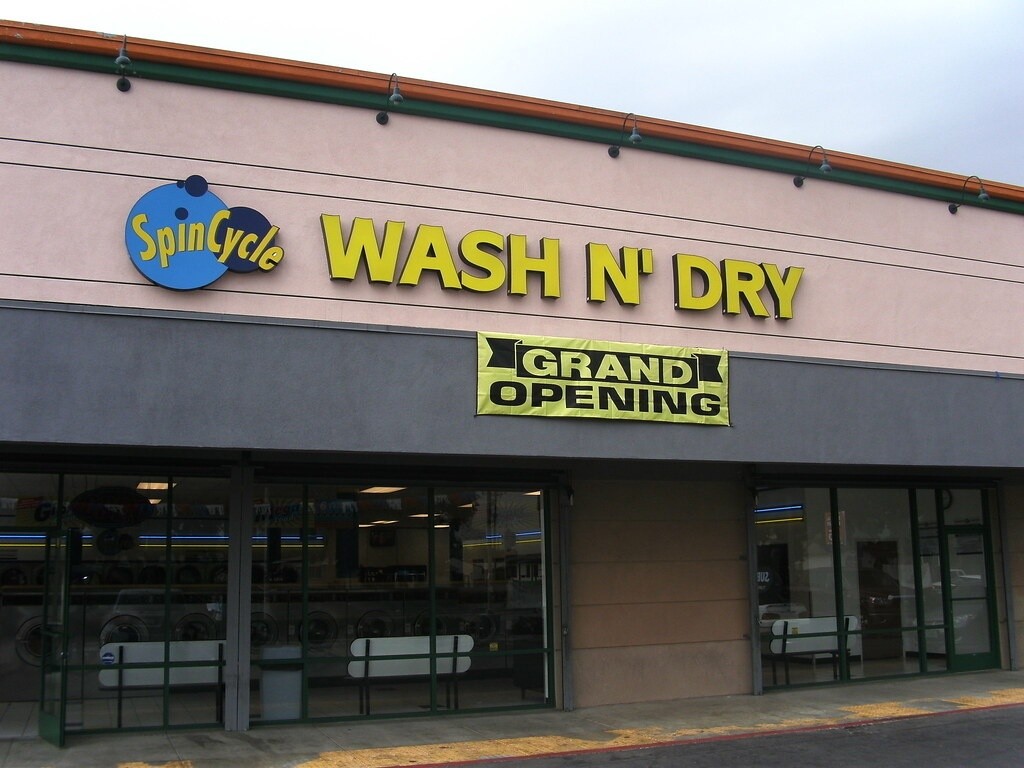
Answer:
[258,644,303,720]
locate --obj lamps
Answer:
[115,34,132,92]
[793,144,832,188]
[948,175,989,214]
[608,112,642,159]
[376,73,403,126]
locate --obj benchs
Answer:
[97,641,224,727]
[343,634,474,715]
[762,615,857,685]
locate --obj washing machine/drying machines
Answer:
[0,580,554,709]
